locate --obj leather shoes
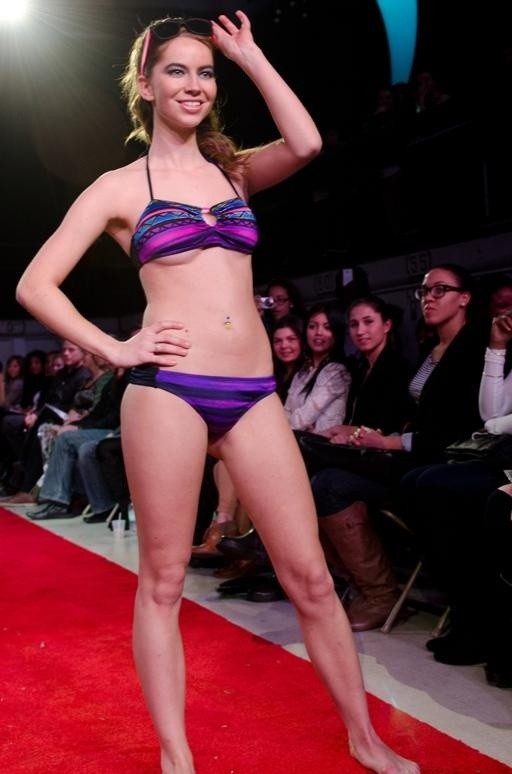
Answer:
[84,508,129,524]
[26,502,75,519]
[9,490,35,503]
[190,520,289,602]
[427,633,488,665]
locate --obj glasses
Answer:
[275,297,288,306]
[140,18,213,76]
[415,283,463,300]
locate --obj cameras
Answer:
[258,296,277,309]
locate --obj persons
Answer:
[15,7,422,774]
[1,311,150,528]
[188,264,512,690]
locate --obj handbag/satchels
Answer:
[445,431,511,464]
[294,429,413,475]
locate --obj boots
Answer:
[318,500,409,632]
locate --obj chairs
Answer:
[81,501,121,524]
[340,507,425,635]
[431,606,451,639]
